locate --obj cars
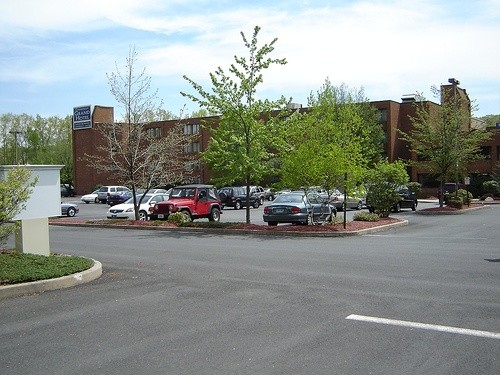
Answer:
[263,191,338,226]
[60,204,79,218]
[216,186,278,210]
[98,185,225,222]
[81,189,102,205]
[274,187,369,211]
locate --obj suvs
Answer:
[366,182,418,212]
[437,183,466,202]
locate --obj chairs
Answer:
[180,191,186,197]
[200,191,207,197]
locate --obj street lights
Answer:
[9,130,21,165]
